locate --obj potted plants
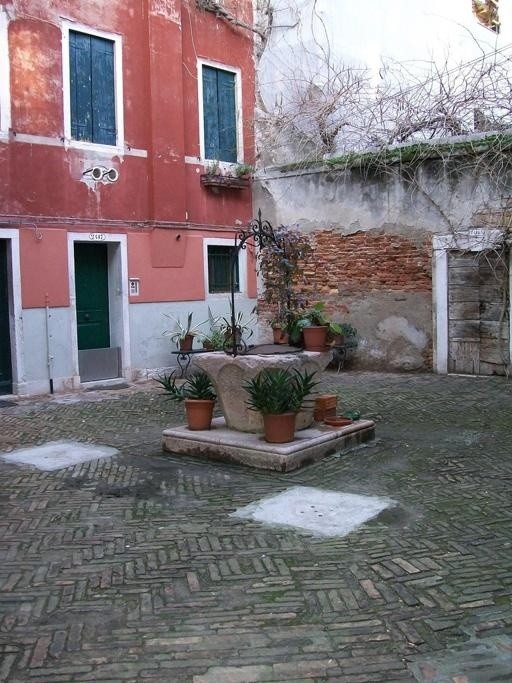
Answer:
[330,324,357,345]
[208,296,256,346]
[286,313,308,347]
[197,330,221,350]
[254,221,317,345]
[240,364,324,443]
[160,312,213,352]
[236,164,257,188]
[151,369,219,430]
[295,301,342,351]
[206,151,221,185]
